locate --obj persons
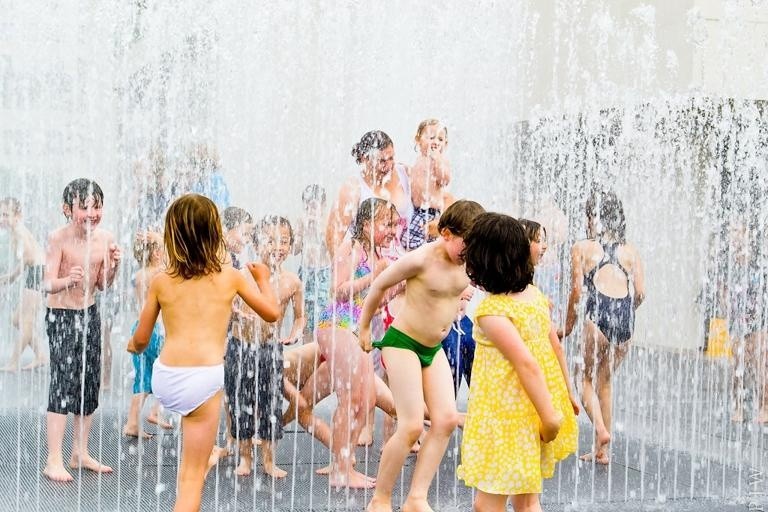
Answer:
[225,214,307,479]
[122,232,174,440]
[289,185,337,344]
[218,206,254,270]
[514,219,548,270]
[554,191,644,465]
[359,200,487,511]
[43,178,122,482]
[316,197,405,489]
[94,277,120,391]
[441,289,477,401]
[462,212,580,512]
[326,131,413,260]
[276,340,398,473]
[401,120,450,251]
[0,196,55,372]
[723,215,767,424]
[126,194,281,512]
